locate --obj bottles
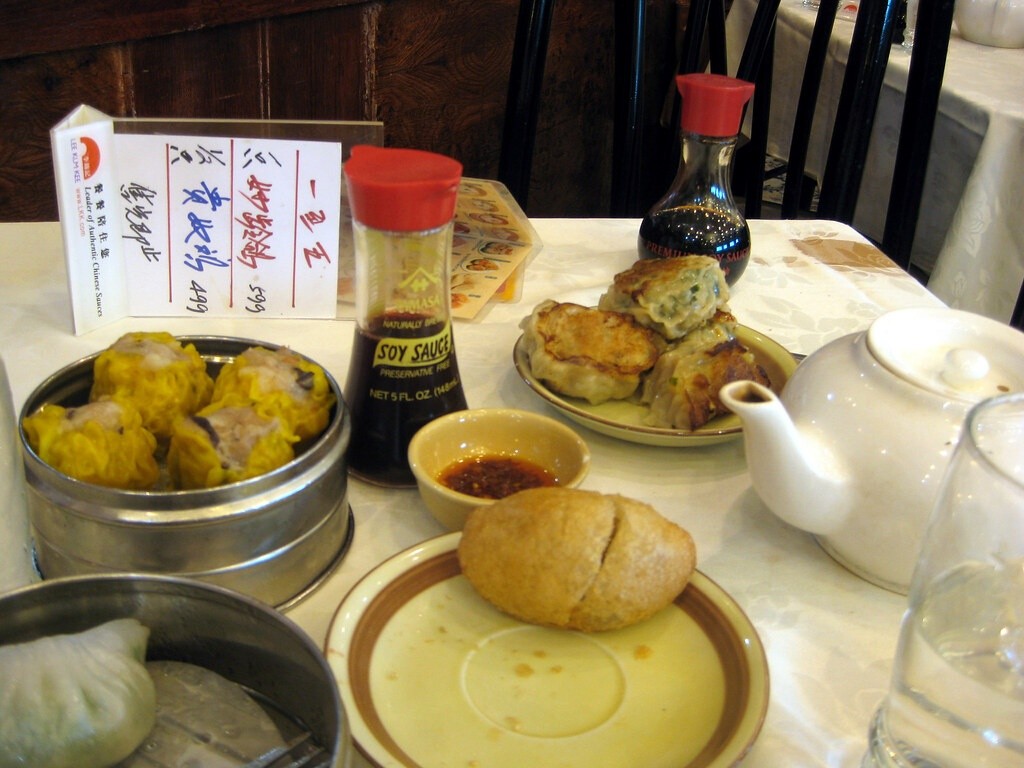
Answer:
[342,145,468,491]
[637,72,756,293]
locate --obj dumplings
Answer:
[515,252,773,431]
[0,616,156,768]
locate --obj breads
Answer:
[459,488,697,630]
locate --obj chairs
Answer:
[496,0,957,274]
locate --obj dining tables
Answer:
[0,217,1024,768]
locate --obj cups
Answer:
[860,393,1024,768]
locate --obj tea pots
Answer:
[718,305,1024,597]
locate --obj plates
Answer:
[322,530,770,768]
[512,304,798,448]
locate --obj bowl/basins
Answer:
[408,408,591,531]
